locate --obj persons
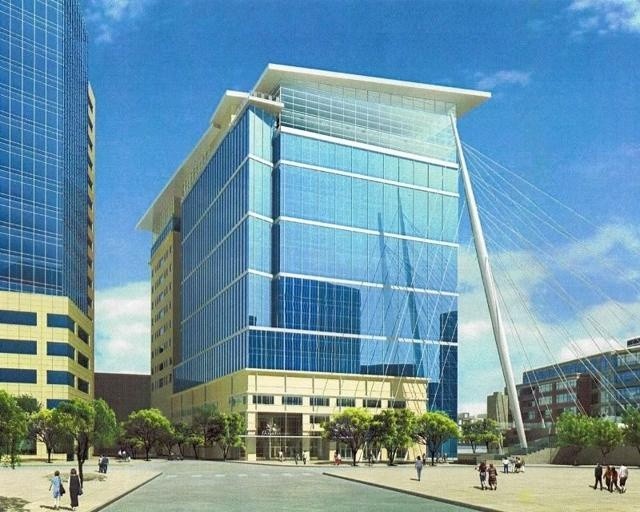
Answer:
[49,471,62,510]
[333,450,341,465]
[415,456,422,481]
[503,457,525,473]
[487,464,497,490]
[475,463,487,489]
[594,463,628,493]
[98,455,103,472]
[68,469,81,510]
[102,455,108,472]
[302,451,307,464]
